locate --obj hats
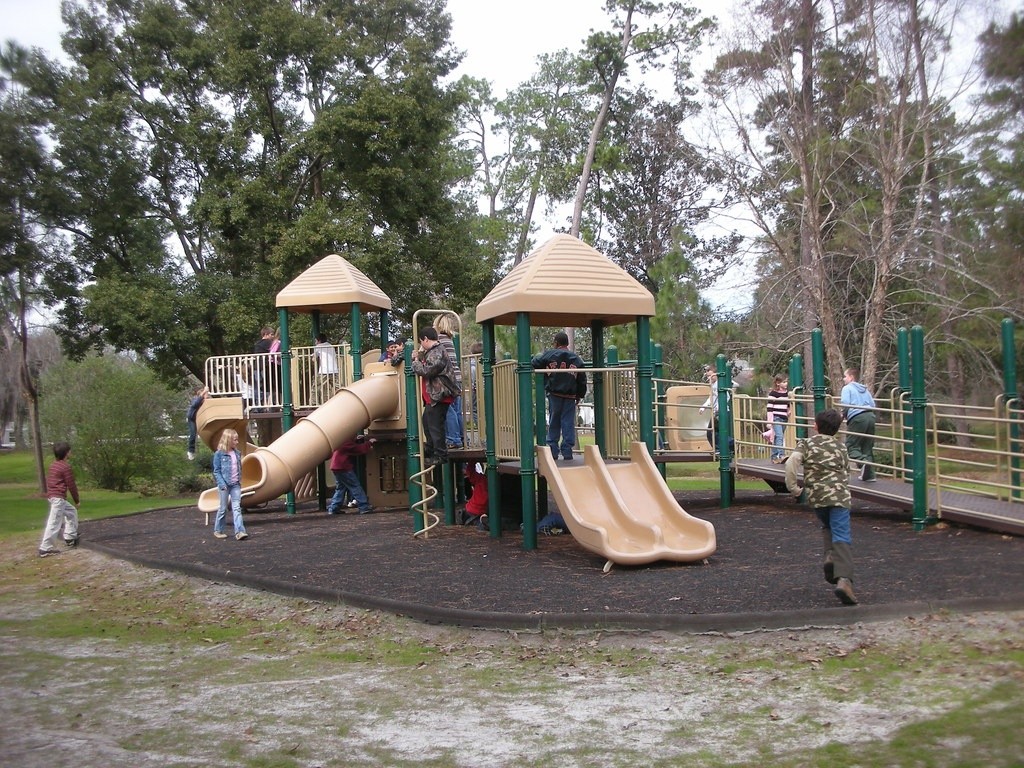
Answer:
[555,332,568,346]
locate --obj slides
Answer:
[536,442,717,566]
[194,376,399,513]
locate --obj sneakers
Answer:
[823,549,838,584]
[834,577,857,606]
[448,442,470,450]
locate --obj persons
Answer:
[531,332,587,460]
[699,365,740,455]
[411,314,470,465]
[378,337,407,367]
[462,461,489,532]
[311,333,340,406]
[39,442,80,558]
[187,386,209,460]
[766,374,792,464]
[236,326,282,413]
[785,409,859,605]
[328,433,377,515]
[466,343,483,430]
[520,510,571,536]
[213,429,248,541]
[840,368,877,482]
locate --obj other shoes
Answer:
[554,458,558,459]
[214,532,227,538]
[465,512,481,525]
[360,508,372,514]
[329,510,345,514]
[771,456,789,464]
[431,457,447,464]
[40,550,60,557]
[455,509,467,525]
[65,540,78,546]
[187,451,195,460]
[564,456,573,460]
[858,476,876,481]
[236,532,248,540]
[710,449,720,455]
